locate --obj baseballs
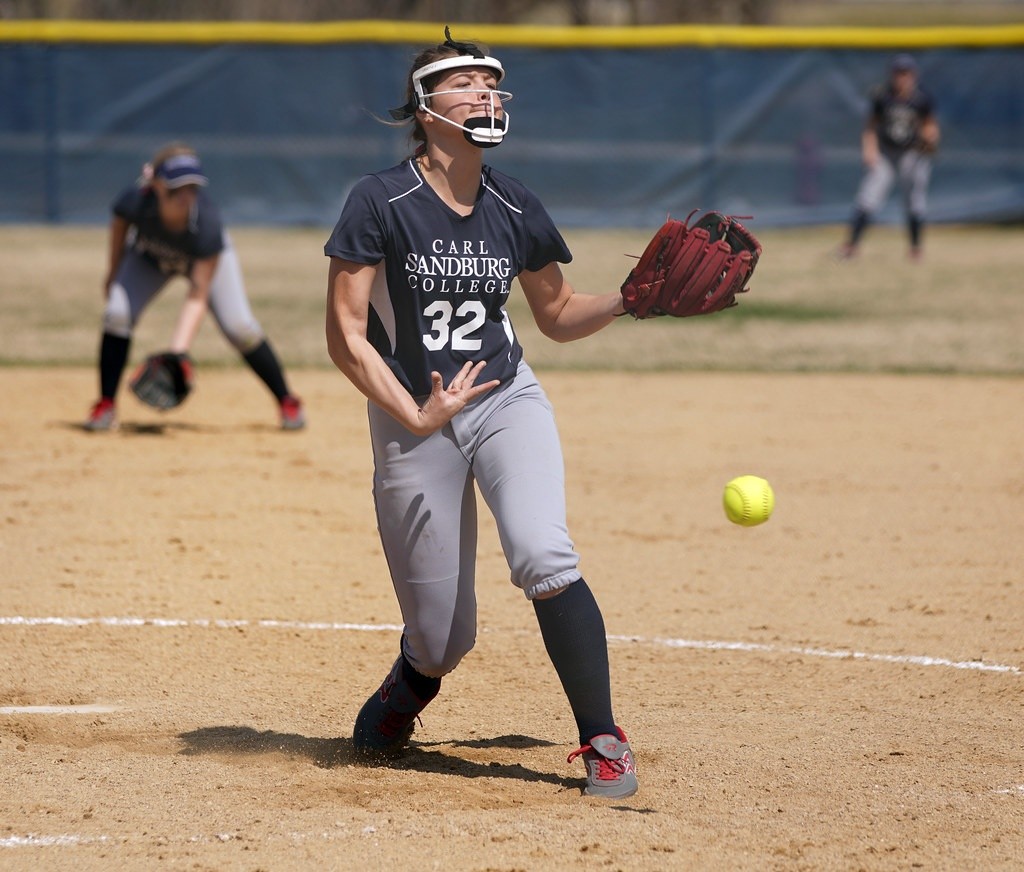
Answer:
[724,476,776,528]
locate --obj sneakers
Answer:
[347,660,425,760]
[86,398,115,431]
[280,396,306,433]
[568,726,640,800]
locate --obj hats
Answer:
[890,56,913,72]
[154,156,212,189]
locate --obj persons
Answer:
[318,44,762,806]
[831,51,946,264]
[82,140,308,437]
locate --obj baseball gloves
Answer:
[129,347,196,413]
[619,209,765,322]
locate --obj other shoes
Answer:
[837,240,853,258]
[907,246,920,260]
